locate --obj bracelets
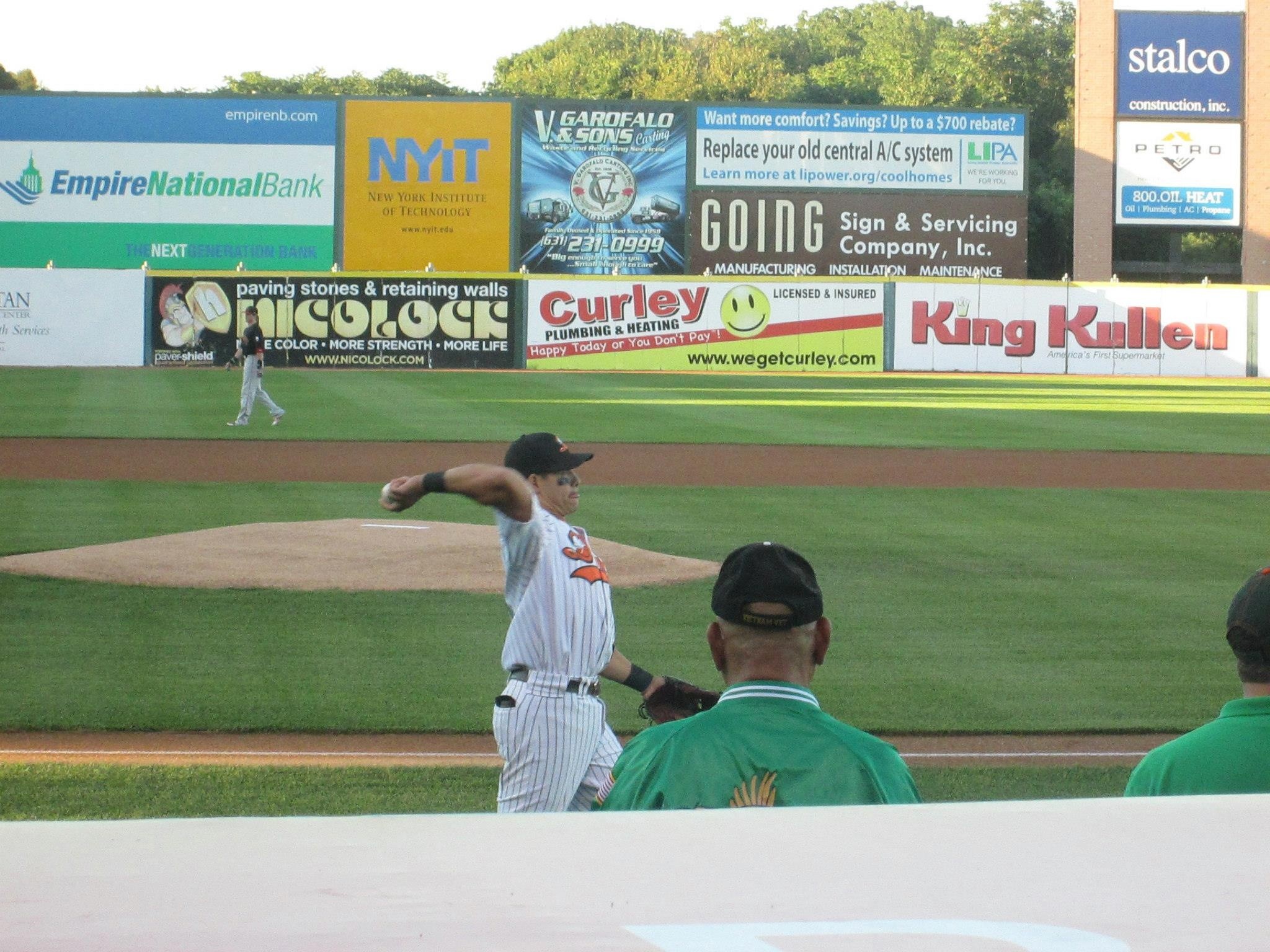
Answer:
[620,662,654,693]
[422,471,450,496]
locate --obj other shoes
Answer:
[227,421,239,426]
[271,415,282,426]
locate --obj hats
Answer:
[711,542,823,629]
[1225,566,1270,648]
[242,306,258,313]
[504,432,593,479]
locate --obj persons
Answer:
[1121,565,1270,797]
[224,305,286,426]
[588,542,923,811]
[379,432,724,811]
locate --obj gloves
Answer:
[257,368,263,377]
[225,361,233,371]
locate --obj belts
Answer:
[510,668,600,695]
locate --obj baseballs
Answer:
[380,482,397,505]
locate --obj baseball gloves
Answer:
[634,676,723,727]
